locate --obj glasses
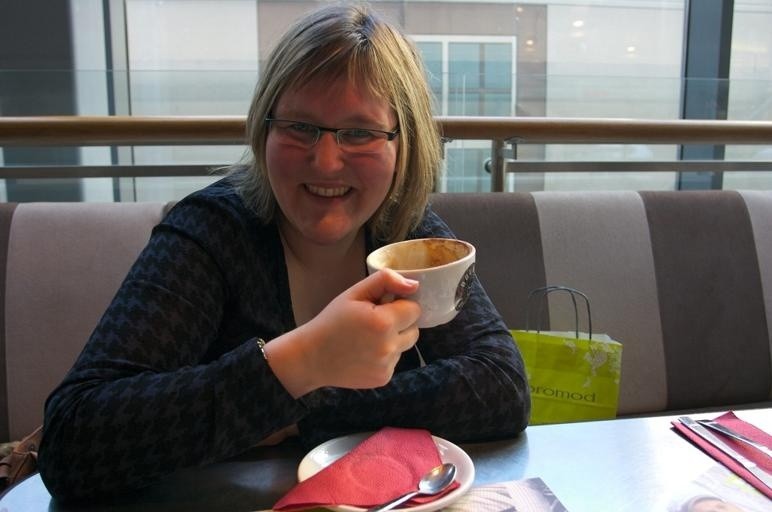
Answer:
[265,118,400,157]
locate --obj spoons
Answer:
[370,462,457,511]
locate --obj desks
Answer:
[0,405,772,512]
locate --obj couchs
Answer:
[2,186,771,455]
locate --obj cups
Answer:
[362,236,478,331]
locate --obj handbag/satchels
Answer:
[0,425,43,486]
[506,286,623,426]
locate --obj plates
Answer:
[293,429,477,512]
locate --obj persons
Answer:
[37,1,532,507]
